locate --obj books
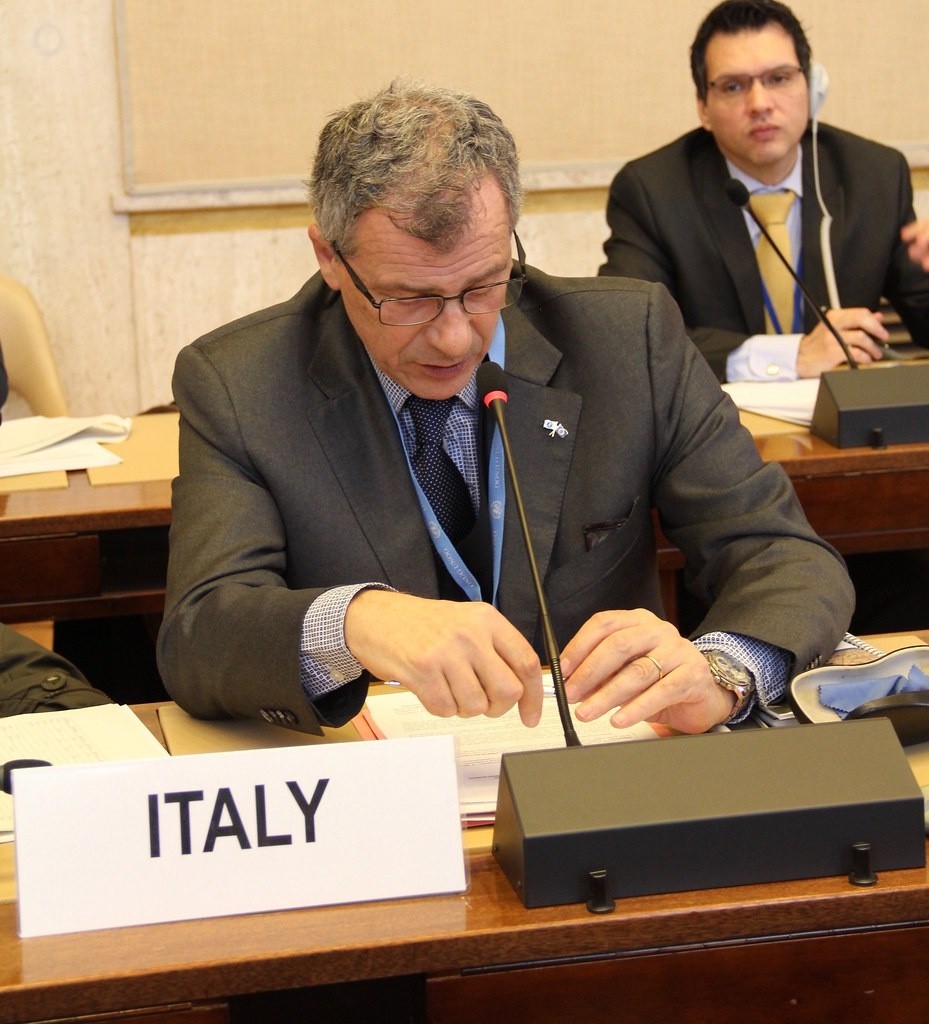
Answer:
[0,413,121,479]
[717,378,820,428]
[751,628,888,728]
[0,701,166,841]
[351,668,734,831]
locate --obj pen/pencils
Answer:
[863,329,889,350]
[383,679,560,695]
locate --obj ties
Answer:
[750,189,794,336]
[404,394,474,546]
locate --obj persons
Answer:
[158,82,856,733]
[597,0,929,389]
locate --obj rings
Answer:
[641,654,663,681]
[847,344,852,351]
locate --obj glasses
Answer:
[332,231,527,327]
[703,66,807,96]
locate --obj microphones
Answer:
[724,180,929,447]
[467,358,929,912]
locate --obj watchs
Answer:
[701,647,754,729]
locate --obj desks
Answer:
[0,626,929,1024]
[0,357,929,623]
